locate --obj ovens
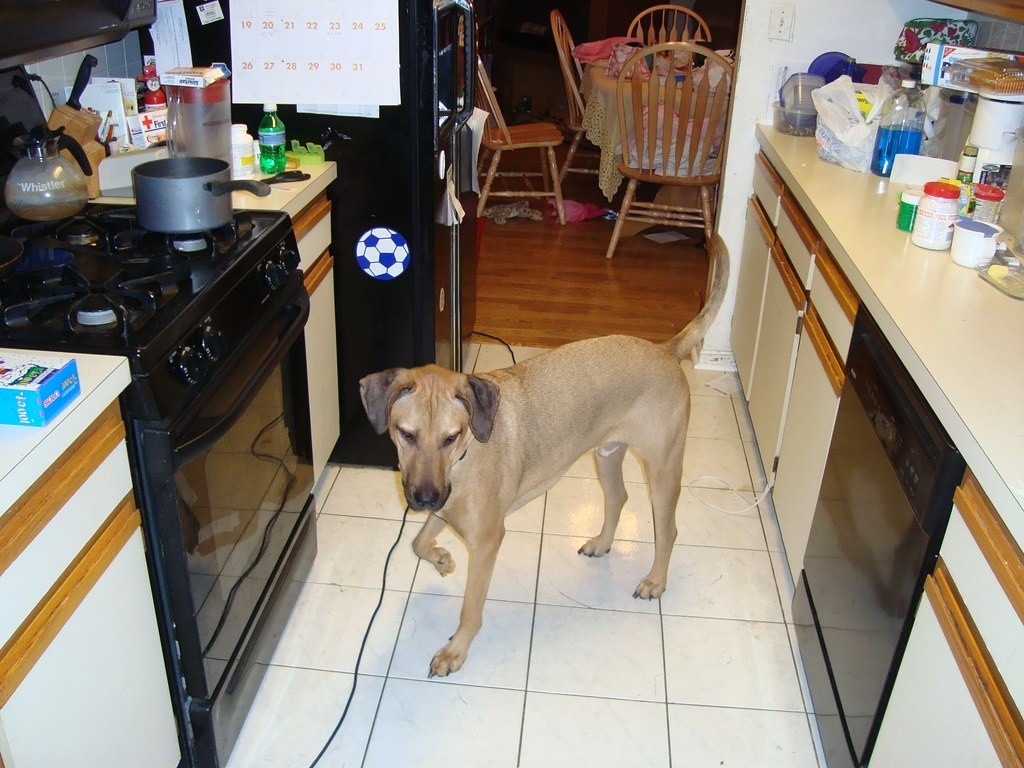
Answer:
[112,226,318,768]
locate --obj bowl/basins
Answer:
[772,72,826,138]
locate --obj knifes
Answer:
[65,54,98,110]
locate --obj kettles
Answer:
[5,126,93,223]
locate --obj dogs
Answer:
[357,232,731,680]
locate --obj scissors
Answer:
[260,170,311,184]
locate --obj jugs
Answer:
[165,78,232,166]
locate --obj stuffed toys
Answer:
[482,200,543,224]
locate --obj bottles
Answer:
[896,190,925,233]
[870,78,927,176]
[257,104,287,176]
[911,181,961,251]
[229,124,257,181]
[956,146,979,185]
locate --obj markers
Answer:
[88,107,99,141]
[107,126,113,141]
[99,110,112,142]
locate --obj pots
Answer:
[131,156,271,234]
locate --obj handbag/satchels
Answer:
[605,41,650,81]
[893,17,978,63]
[811,74,941,175]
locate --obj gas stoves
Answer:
[0,202,293,374]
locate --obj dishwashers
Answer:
[788,298,967,768]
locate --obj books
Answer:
[64,82,127,144]
[92,78,138,116]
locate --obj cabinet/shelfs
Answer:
[0,398,190,767]
[724,154,1024,768]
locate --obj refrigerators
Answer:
[182,1,476,471]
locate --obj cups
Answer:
[949,219,1004,270]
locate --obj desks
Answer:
[558,46,733,211]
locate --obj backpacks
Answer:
[692,48,735,97]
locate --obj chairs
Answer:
[549,4,734,260]
[474,47,565,228]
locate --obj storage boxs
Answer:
[780,74,826,123]
[126,108,168,150]
[0,350,80,427]
[920,41,1024,94]
[771,101,818,136]
